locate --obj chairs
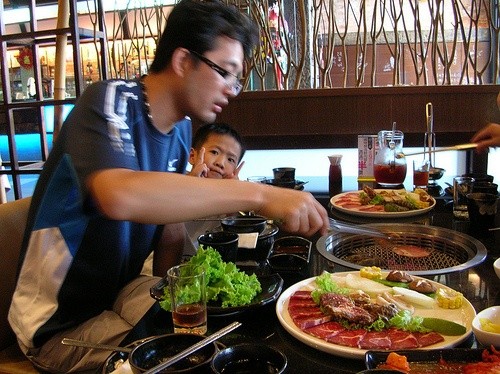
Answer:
[0,196,69,374]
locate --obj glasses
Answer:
[187,49,243,97]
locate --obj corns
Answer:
[360,266,381,278]
[436,287,463,309]
[416,201,430,209]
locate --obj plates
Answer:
[275,271,477,360]
[102,335,156,374]
[149,266,283,315]
[330,189,436,218]
[365,348,500,374]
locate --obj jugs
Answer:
[374,121,407,188]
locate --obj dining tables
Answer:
[102,192,500,374]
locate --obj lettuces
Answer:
[312,270,433,333]
[160,243,262,312]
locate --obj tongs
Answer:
[328,216,396,241]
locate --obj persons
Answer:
[470,91,500,155]
[8,0,329,374]
[152,122,246,280]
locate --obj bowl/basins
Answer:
[130,174,500,374]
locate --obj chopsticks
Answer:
[142,321,242,374]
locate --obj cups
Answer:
[413,160,430,193]
[453,176,475,220]
[273,168,295,188]
[196,230,239,264]
[247,176,267,217]
[167,264,208,335]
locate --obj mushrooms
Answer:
[386,271,414,282]
[409,278,437,293]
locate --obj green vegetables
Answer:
[360,191,416,213]
